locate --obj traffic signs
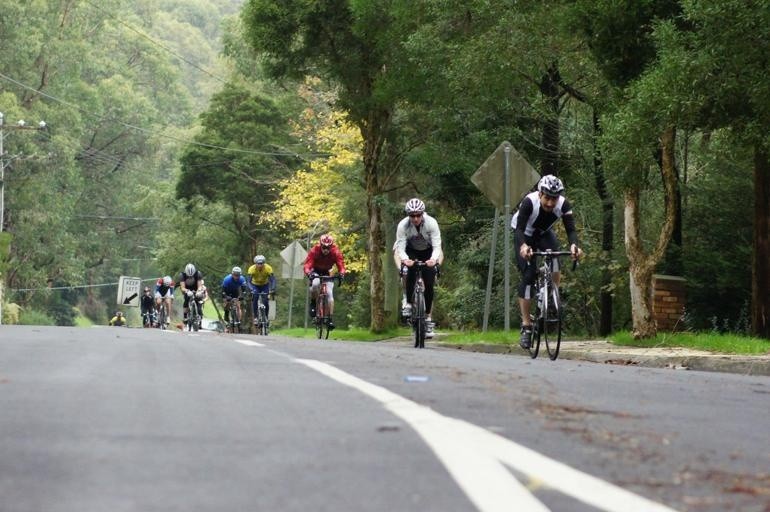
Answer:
[121,276,140,306]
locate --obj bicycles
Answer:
[307,273,343,339]
[398,258,441,348]
[525,250,577,361]
[141,290,276,336]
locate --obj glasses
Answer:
[322,245,331,250]
[409,212,423,217]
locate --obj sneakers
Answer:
[520,325,532,349]
[310,304,316,317]
[327,321,335,330]
[402,299,411,317]
[424,321,434,338]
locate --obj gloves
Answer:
[339,276,344,281]
[308,274,315,280]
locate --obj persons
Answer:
[511,174,584,350]
[247,255,275,328]
[109,263,208,330]
[392,198,444,339]
[222,267,247,321]
[304,235,344,329]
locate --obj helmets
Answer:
[538,175,564,192]
[117,312,122,317]
[253,255,265,264]
[232,266,242,275]
[163,276,172,287]
[185,263,196,277]
[405,198,425,214]
[320,234,333,246]
